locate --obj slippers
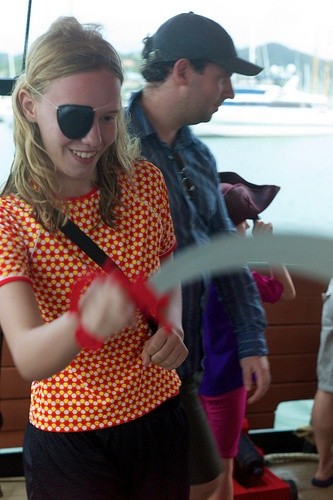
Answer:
[311,477,333,487]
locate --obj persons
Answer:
[197,183,296,500]
[123,10,270,500]
[0,18,226,500]
[311,277,333,487]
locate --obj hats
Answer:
[217,171,281,225]
[139,11,264,76]
